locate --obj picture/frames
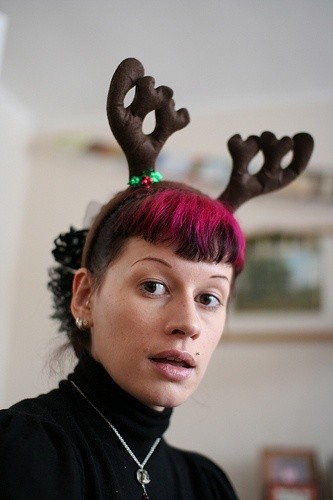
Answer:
[260,447,319,500]
[214,226,333,340]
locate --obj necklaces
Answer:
[70,380,162,500]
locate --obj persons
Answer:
[0,171,314,500]
